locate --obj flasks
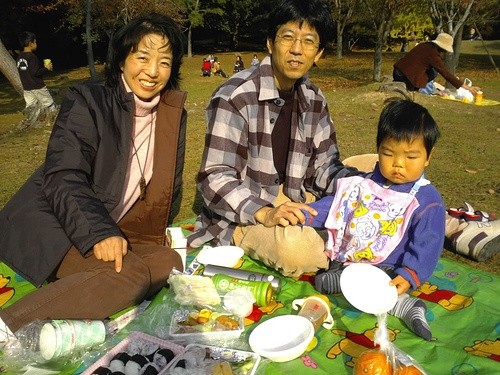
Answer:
[203,264,281,294]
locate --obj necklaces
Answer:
[131,109,152,200]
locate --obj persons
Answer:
[285,85,445,342]
[192,0,500,279]
[250,54,259,66]
[469,28,476,40]
[0,9,187,355]
[16,34,57,115]
[200,54,229,78]
[393,32,481,97]
[234,56,245,72]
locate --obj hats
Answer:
[432,33,454,53]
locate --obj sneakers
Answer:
[452,220,500,260]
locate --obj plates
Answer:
[340,262,398,314]
[169,312,245,340]
[161,343,261,375]
[79,331,184,375]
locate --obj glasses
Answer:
[275,33,321,48]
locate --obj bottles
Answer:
[474,90,483,104]
[203,273,272,307]
[292,294,334,331]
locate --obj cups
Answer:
[39,320,105,360]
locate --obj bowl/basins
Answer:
[248,315,315,363]
[196,246,244,268]
